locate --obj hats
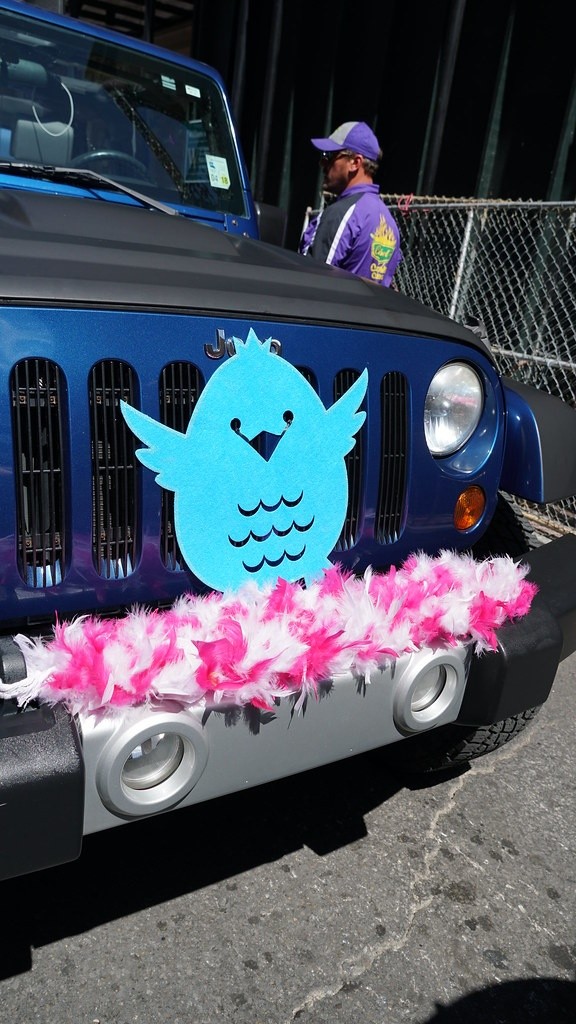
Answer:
[311,121,378,161]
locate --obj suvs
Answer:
[1,0,565,880]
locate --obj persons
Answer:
[298,117,400,287]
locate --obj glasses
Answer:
[321,152,352,164]
